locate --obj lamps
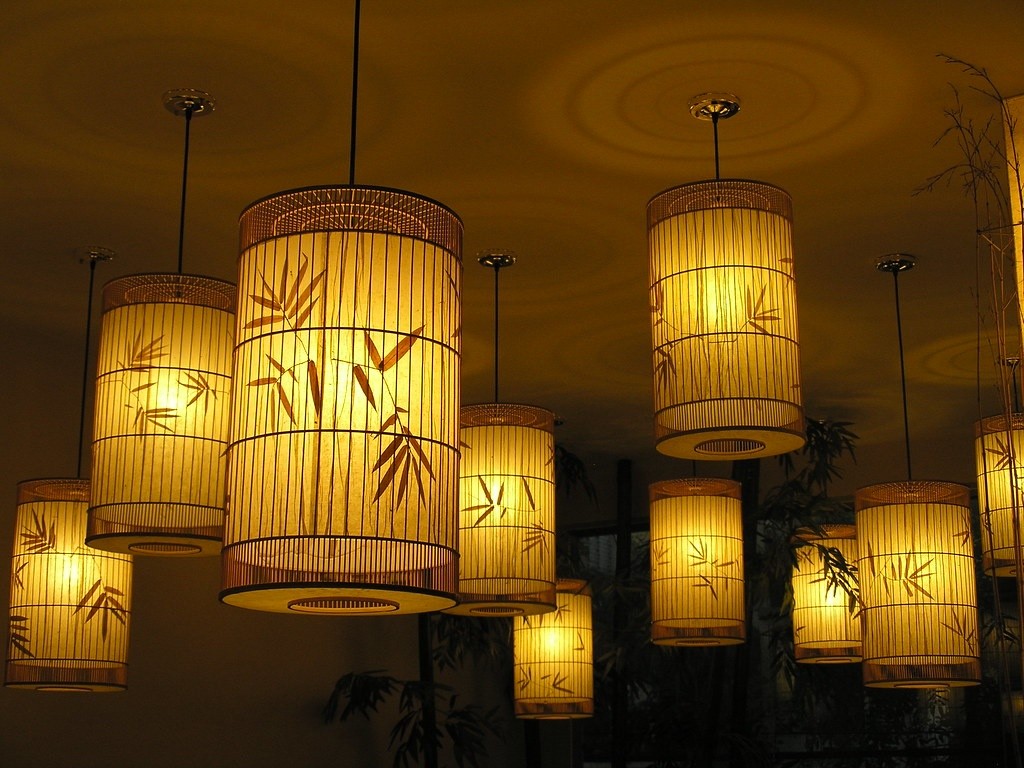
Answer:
[84,109,237,560]
[853,265,983,690]
[439,267,562,617]
[789,478,863,664]
[644,112,809,462]
[512,579,594,721]
[217,1,460,617]
[1,261,133,693]
[649,461,749,646]
[973,365,1024,578]
